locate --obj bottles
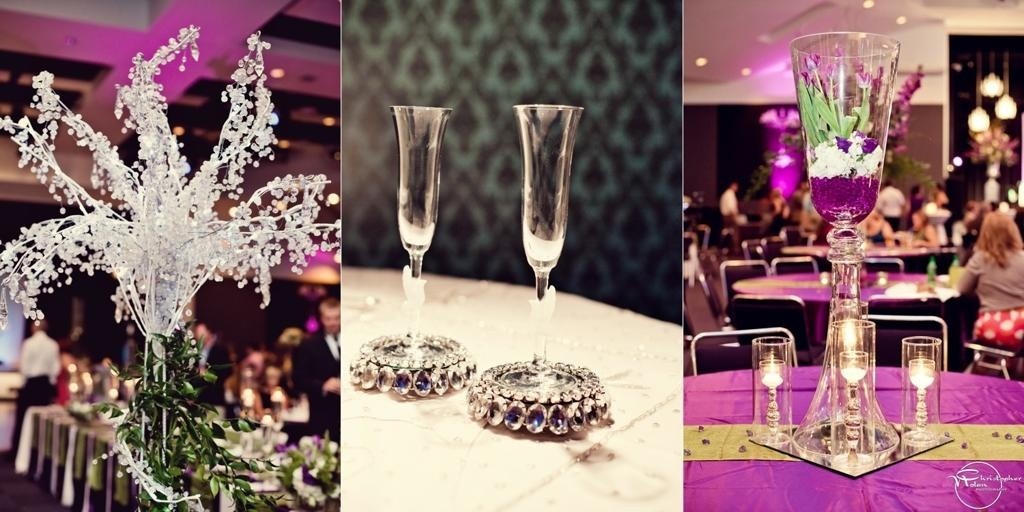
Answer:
[928,257,937,285]
[949,255,961,287]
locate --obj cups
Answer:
[828,299,870,421]
[751,336,794,445]
[902,336,942,446]
[828,319,876,469]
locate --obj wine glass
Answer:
[501,104,586,394]
[376,104,455,360]
[793,31,902,461]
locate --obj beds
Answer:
[16,404,140,510]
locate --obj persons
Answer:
[719,181,1022,382]
[2,294,341,485]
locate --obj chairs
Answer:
[731,294,826,361]
[779,227,801,246]
[961,309,1016,381]
[691,326,798,375]
[864,258,904,272]
[720,260,772,313]
[869,296,943,330]
[859,314,948,372]
[736,224,761,255]
[760,236,784,262]
[683,232,698,277]
[771,256,819,275]
[698,222,709,258]
[742,240,761,259]
